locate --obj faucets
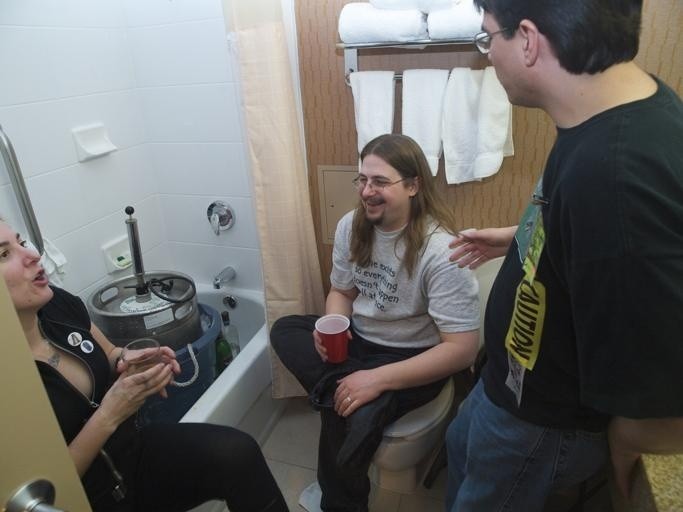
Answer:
[214,268,236,289]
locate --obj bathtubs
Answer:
[171,285,291,446]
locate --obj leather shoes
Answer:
[333,391,399,473]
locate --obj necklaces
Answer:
[32,338,61,366]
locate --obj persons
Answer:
[270,134,481,511]
[447,0,682,510]
[0,217,289,511]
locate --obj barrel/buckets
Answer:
[145,303,221,421]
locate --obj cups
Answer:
[120,338,161,391]
[315,313,350,363]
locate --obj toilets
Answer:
[371,378,455,495]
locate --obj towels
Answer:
[338,0,484,42]
[348,69,516,185]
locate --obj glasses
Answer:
[352,175,406,194]
[474,16,544,57]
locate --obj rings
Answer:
[346,396,353,404]
